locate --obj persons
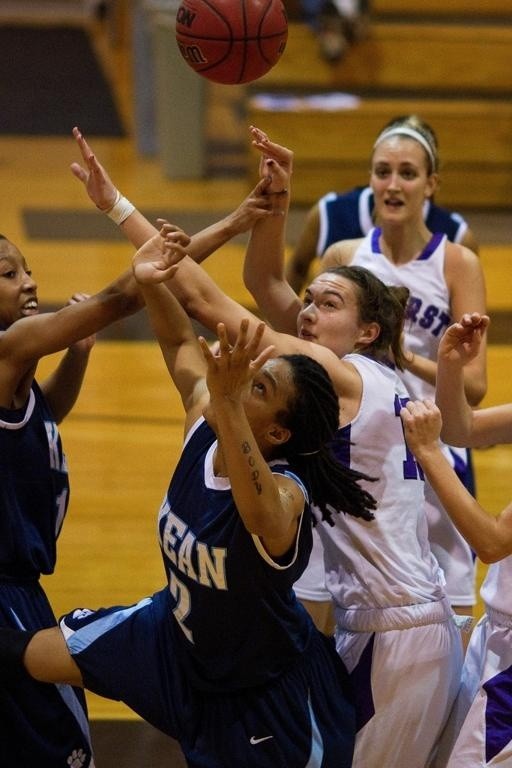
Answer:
[68,126,465,768]
[0,172,287,765]
[284,116,479,294]
[320,123,492,653]
[401,314,512,768]
[27,218,377,768]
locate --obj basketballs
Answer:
[175,0,288,85]
